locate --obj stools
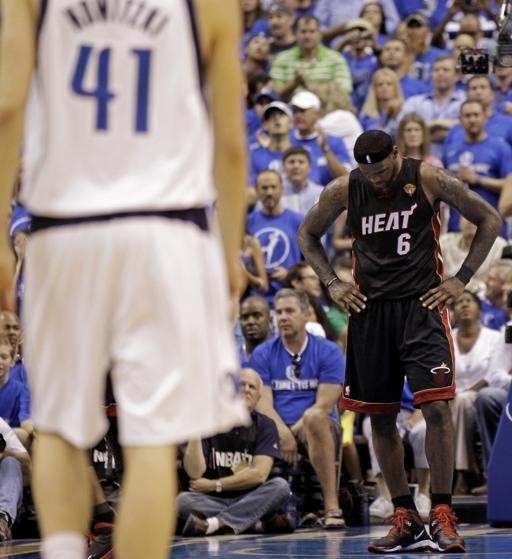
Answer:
[296,460,342,527]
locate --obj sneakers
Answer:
[366,505,430,554]
[266,511,296,534]
[414,492,433,523]
[1,517,14,543]
[368,495,395,520]
[86,506,118,559]
[181,513,210,537]
[425,503,466,553]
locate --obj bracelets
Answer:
[455,265,474,284]
[326,276,339,288]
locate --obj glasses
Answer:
[291,353,302,379]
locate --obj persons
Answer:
[358,374,433,520]
[0,190,37,559]
[444,291,503,496]
[80,370,126,557]
[475,258,512,330]
[441,101,512,241]
[469,289,512,496]
[241,0,379,179]
[381,0,512,131]
[433,209,502,309]
[394,112,447,240]
[171,368,292,537]
[239,167,316,288]
[297,132,512,553]
[279,145,335,264]
[246,289,349,532]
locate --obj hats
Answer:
[290,90,322,113]
[345,17,372,34]
[263,100,293,120]
[252,87,282,104]
[405,12,430,28]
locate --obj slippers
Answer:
[322,506,347,530]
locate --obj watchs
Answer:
[215,479,223,493]
